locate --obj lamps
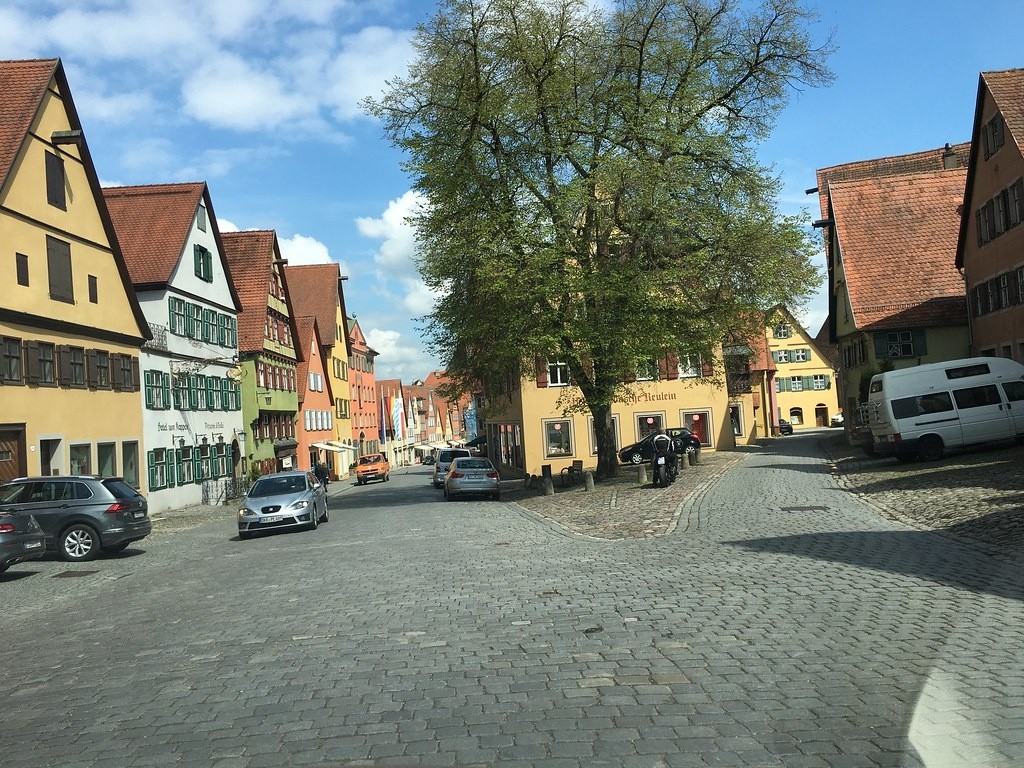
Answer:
[256,390,271,404]
[173,434,186,448]
[830,356,842,379]
[241,457,247,474]
[212,432,223,444]
[169,351,248,389]
[195,433,208,445]
[234,428,247,442]
[251,418,261,440]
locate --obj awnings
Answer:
[415,440,468,450]
[311,440,358,454]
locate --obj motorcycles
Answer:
[656,451,676,488]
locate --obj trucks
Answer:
[356,453,390,485]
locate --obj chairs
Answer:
[374,456,380,462]
[292,478,303,488]
[360,458,368,464]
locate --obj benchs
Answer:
[561,460,584,479]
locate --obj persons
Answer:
[315,459,330,492]
[652,427,679,488]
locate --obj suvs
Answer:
[0,475,153,562]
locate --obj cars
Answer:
[0,508,47,573]
[422,455,436,466]
[236,470,330,539]
[779,419,793,435]
[831,412,846,426]
[444,456,502,501]
[617,427,702,465]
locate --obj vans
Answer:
[433,449,471,490]
[860,357,1024,458]
